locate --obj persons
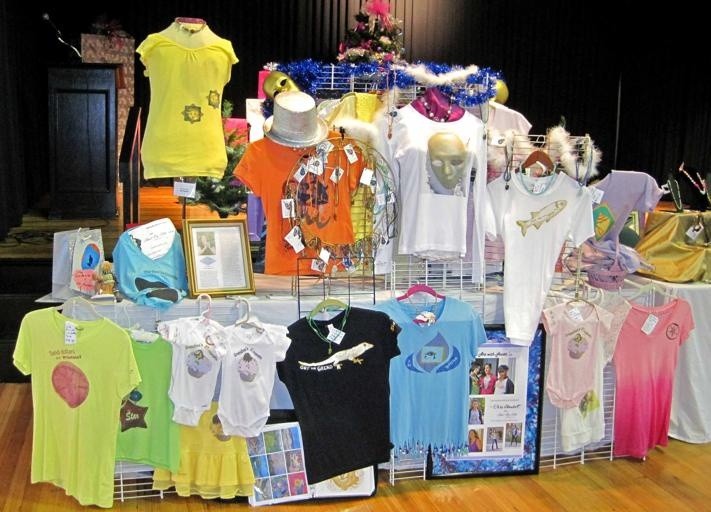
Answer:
[493,364,514,394]
[198,234,216,255]
[510,423,519,446]
[428,130,468,190]
[491,429,500,450]
[468,428,482,452]
[467,399,483,424]
[388,83,494,286]
[468,362,481,395]
[478,363,497,395]
[137,17,240,180]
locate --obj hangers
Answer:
[542,262,684,321]
[50,269,257,344]
[301,276,448,330]
[503,134,565,180]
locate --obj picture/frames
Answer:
[182,218,255,299]
[423,324,544,480]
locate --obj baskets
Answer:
[588,234,628,291]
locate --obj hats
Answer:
[263,89,330,149]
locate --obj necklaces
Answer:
[668,179,683,210]
[308,303,351,356]
[420,94,452,123]
[576,134,594,197]
[501,129,515,189]
[175,18,207,33]
[406,290,438,324]
[682,168,706,195]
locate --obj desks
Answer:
[62,273,711,491]
